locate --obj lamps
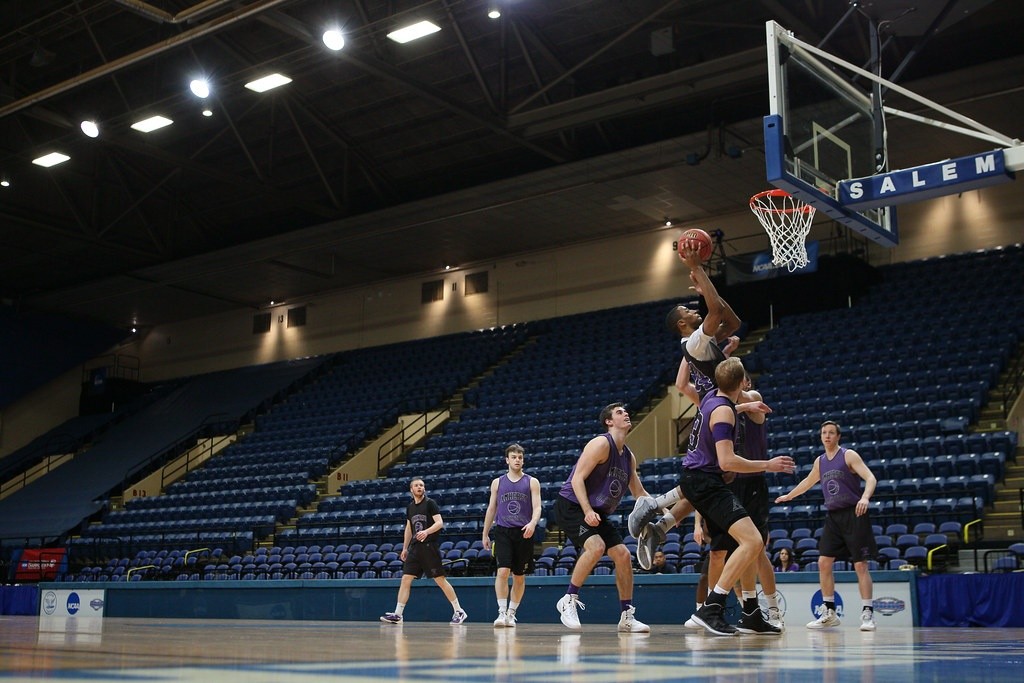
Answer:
[129,326,138,333]
[443,261,450,269]
[1,6,508,191]
[263,296,274,306]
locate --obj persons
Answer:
[651,551,676,574]
[379,478,468,625]
[775,421,878,631]
[551,402,651,632]
[774,546,798,573]
[481,444,542,628]
[629,248,800,635]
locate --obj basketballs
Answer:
[678,228,713,263]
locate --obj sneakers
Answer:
[690,600,740,637]
[859,608,876,631]
[628,495,660,539]
[637,522,667,570]
[684,618,703,630]
[618,630,651,646]
[556,594,584,629]
[807,604,840,629]
[449,611,467,625]
[616,606,649,633]
[505,607,517,627]
[379,611,403,623]
[736,607,781,635]
[766,610,784,630]
[494,607,506,628]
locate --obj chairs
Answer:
[1,240,1024,589]
